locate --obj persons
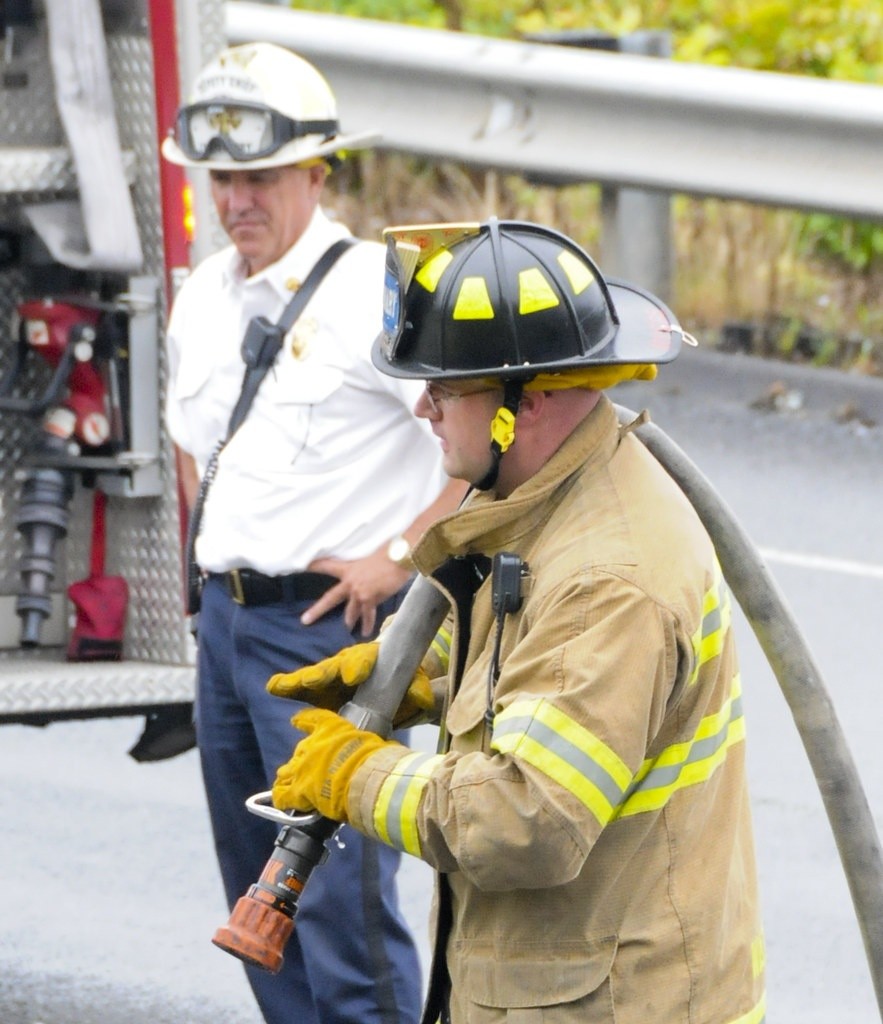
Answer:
[263,219,775,1023]
[166,45,460,1023]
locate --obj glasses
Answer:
[425,380,497,405]
[172,102,339,161]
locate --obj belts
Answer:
[203,568,336,607]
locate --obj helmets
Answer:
[159,42,341,170]
[369,212,685,380]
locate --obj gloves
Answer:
[270,706,401,839]
[268,639,437,725]
